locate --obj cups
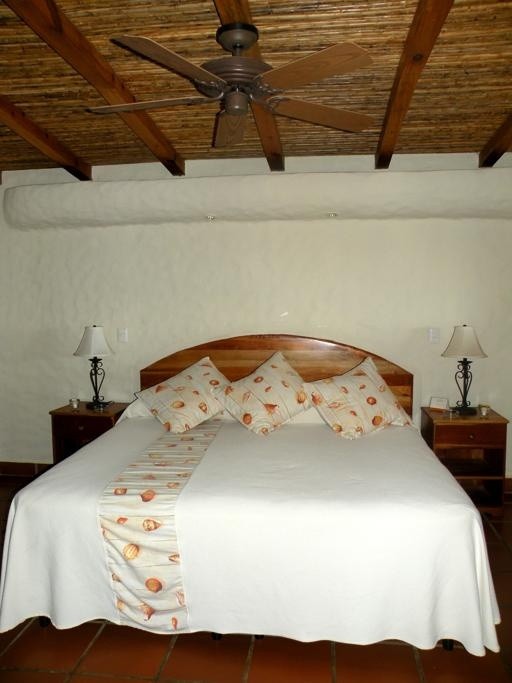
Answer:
[478,404,490,418]
[68,398,81,411]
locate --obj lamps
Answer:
[74,324,115,410]
[442,325,488,415]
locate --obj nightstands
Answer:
[422,405,510,509]
[49,402,129,465]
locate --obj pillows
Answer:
[134,349,418,439]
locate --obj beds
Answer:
[8,334,480,651]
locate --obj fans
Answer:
[89,23,374,150]
